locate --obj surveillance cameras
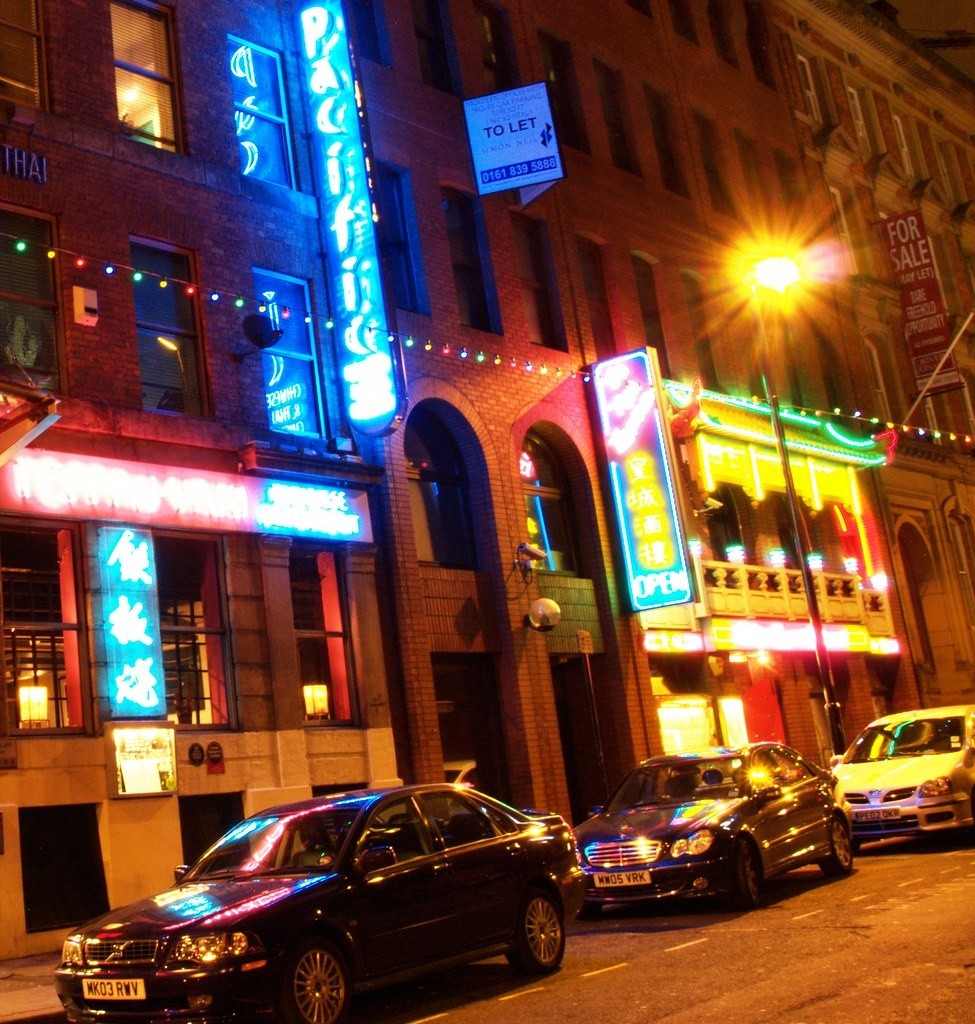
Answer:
[518,543,548,560]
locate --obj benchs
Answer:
[694,770,739,798]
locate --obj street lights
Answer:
[740,246,847,756]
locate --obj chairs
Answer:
[290,820,331,866]
[664,774,696,801]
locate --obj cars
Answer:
[571,739,856,919]
[823,703,975,849]
[52,779,587,1023]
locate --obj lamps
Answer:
[331,438,352,454]
[303,685,330,720]
[525,598,561,632]
[19,686,50,728]
